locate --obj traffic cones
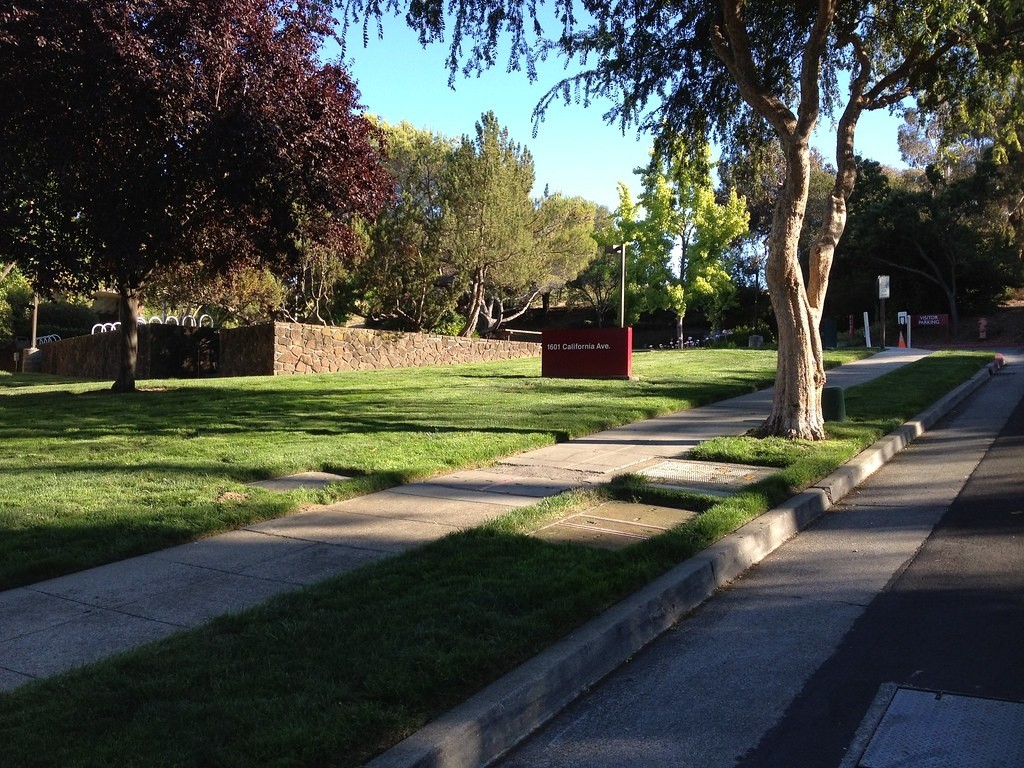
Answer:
[898,330,905,347]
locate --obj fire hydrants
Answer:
[977,317,988,340]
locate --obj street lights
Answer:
[604,244,625,328]
